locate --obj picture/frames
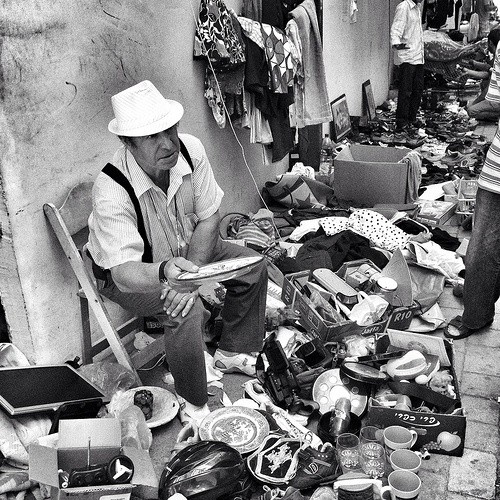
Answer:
[329,79,378,143]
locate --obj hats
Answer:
[108,80,184,137]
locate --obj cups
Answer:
[374,278,398,304]
[336,426,422,500]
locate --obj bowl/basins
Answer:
[341,362,388,395]
[317,411,362,447]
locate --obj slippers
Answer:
[444,315,494,340]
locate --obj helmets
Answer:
[157,441,249,500]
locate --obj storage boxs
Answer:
[28,416,160,494]
[334,144,415,207]
[279,260,419,345]
[368,334,467,458]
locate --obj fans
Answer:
[459,12,480,46]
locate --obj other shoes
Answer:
[364,101,491,185]
[213,349,269,377]
[175,389,210,428]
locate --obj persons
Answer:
[444,1,500,339]
[391,0,426,133]
[85,80,271,431]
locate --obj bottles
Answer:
[321,134,331,162]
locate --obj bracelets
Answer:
[158,260,168,285]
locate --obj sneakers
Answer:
[255,485,303,500]
[289,444,343,488]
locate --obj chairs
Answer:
[43,181,230,390]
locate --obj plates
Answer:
[312,369,367,418]
[114,386,180,428]
[199,406,270,455]
[176,256,264,286]
